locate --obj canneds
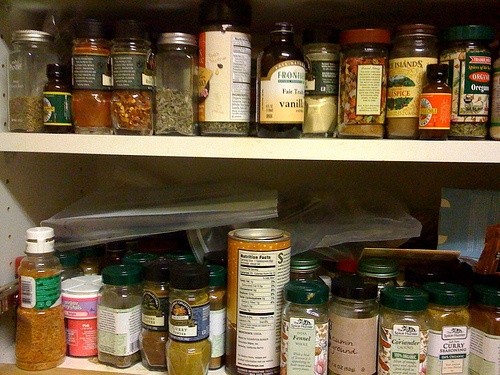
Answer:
[6,21,500,141]
[57,228,500,375]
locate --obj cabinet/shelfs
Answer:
[1,128,500,375]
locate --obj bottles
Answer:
[56,241,226,374]
[278,254,499,375]
[257,20,500,140]
[17,226,67,372]
[7,14,198,135]
[198,1,251,135]
